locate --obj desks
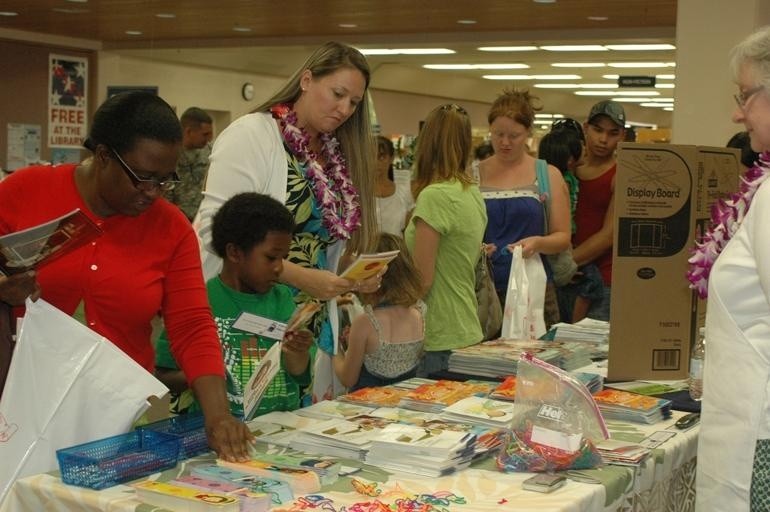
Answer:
[13,377,701,511]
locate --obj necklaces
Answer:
[684,149,770,299]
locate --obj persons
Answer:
[191,41,388,405]
[155,192,313,419]
[163,107,212,223]
[333,90,636,390]
[694,25,770,512]
[2,89,257,462]
[723,131,765,175]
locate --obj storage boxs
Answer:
[606,141,743,385]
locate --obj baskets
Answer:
[135,409,245,461]
[56,427,184,490]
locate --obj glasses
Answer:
[733,85,764,107]
[109,146,182,192]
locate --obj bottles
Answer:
[688,326,705,402]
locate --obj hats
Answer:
[588,100,625,128]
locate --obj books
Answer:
[128,315,694,511]
